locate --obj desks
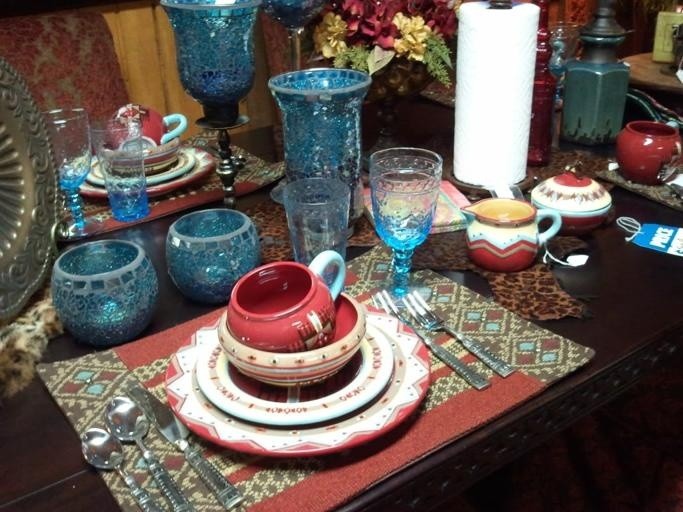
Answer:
[0,95,683,511]
[620,52,683,93]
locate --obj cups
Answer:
[49,238,155,349]
[166,210,261,299]
[267,65,367,238]
[615,120,682,186]
[88,115,151,223]
[110,105,187,148]
[529,161,614,237]
[283,177,350,274]
[228,250,344,353]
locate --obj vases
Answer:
[328,58,433,146]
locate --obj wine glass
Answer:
[368,147,441,295]
[547,20,585,107]
[43,107,106,239]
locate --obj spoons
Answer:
[79,427,158,511]
[102,396,191,509]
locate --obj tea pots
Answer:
[458,199,559,275]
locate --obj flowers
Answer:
[312,1,458,90]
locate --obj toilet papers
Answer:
[453,1,541,188]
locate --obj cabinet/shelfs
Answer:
[258,13,375,123]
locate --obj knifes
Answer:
[127,384,242,509]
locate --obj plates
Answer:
[196,324,391,425]
[85,151,196,187]
[75,144,211,199]
[164,309,427,460]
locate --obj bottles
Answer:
[525,28,558,169]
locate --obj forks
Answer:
[370,290,491,392]
[402,292,518,379]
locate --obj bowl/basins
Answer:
[98,134,180,176]
[217,290,367,387]
